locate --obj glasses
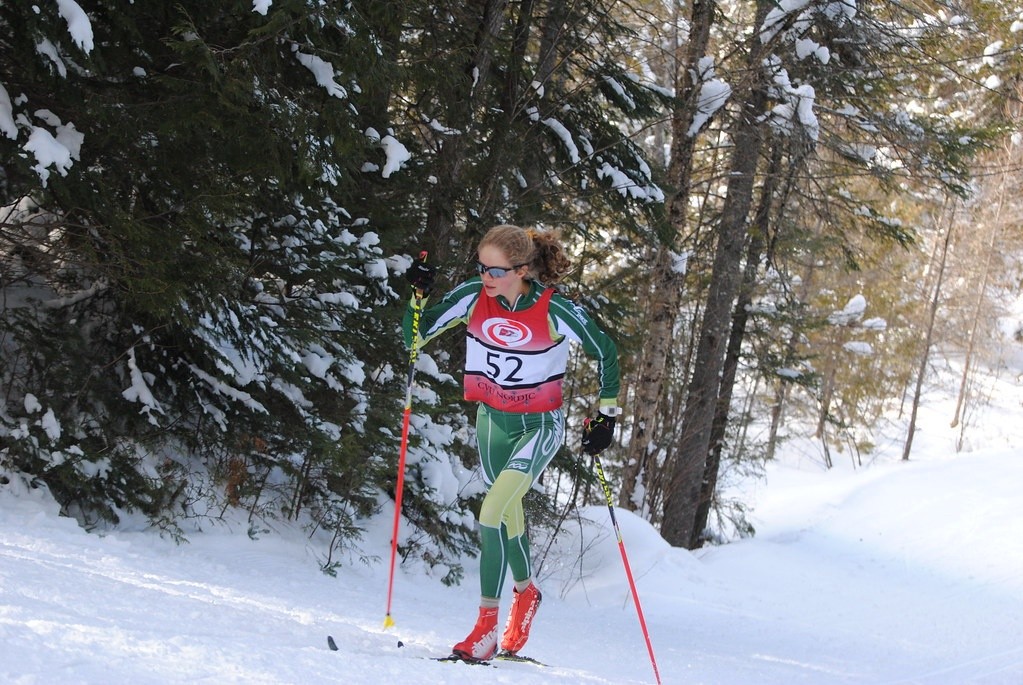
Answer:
[476,260,527,278]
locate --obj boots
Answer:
[501,581,543,654]
[453,606,500,661]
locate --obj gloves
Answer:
[405,258,437,298]
[582,410,616,456]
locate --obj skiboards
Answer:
[321,632,538,666]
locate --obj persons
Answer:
[403,225,623,662]
